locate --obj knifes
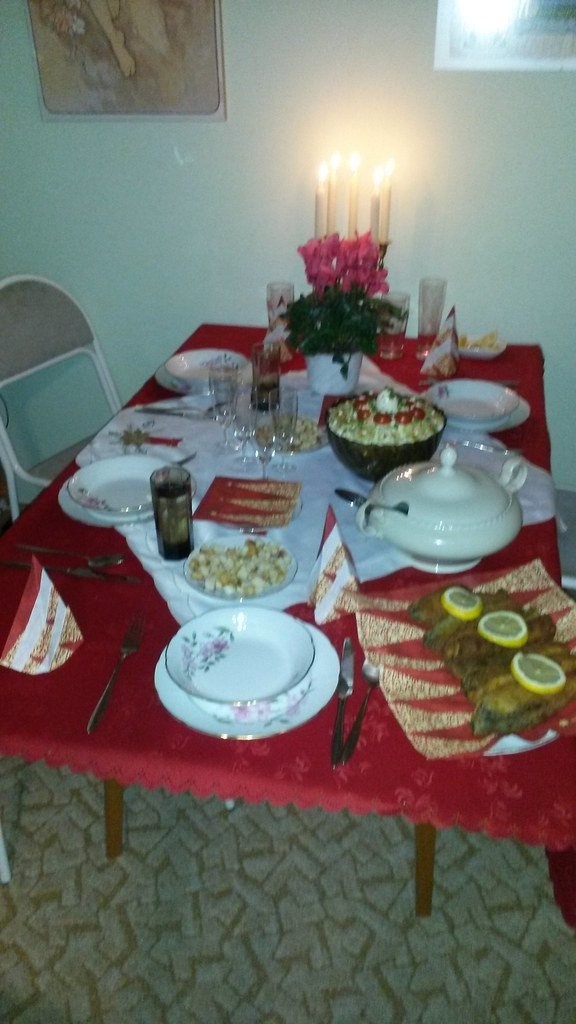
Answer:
[0,562,142,586]
[331,636,354,771]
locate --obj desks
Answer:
[0,322,576,918]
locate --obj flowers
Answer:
[276,234,405,380]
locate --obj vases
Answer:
[305,352,363,396]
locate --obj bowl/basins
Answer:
[165,348,248,384]
[325,393,447,483]
[355,444,527,574]
[460,323,509,361]
[426,379,520,422]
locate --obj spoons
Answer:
[340,657,380,765]
[17,544,126,567]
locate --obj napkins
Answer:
[347,559,576,761]
[309,504,364,625]
[420,305,460,378]
[1,552,84,675]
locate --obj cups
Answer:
[415,277,448,360]
[266,281,294,332]
[150,466,194,560]
[378,290,411,361]
[250,342,281,410]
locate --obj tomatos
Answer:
[352,391,426,424]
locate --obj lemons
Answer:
[511,651,566,695]
[442,587,481,621]
[477,610,529,648]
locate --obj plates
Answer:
[217,495,303,532]
[154,357,252,395]
[155,620,340,740]
[57,476,196,527]
[420,392,531,432]
[67,455,192,512]
[183,535,298,600]
[165,606,315,706]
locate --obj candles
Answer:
[316,184,327,239]
[348,175,360,239]
[325,168,339,237]
[379,180,391,241]
[371,195,381,243]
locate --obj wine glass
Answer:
[207,369,299,480]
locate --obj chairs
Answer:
[1,277,120,521]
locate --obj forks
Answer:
[85,614,142,734]
